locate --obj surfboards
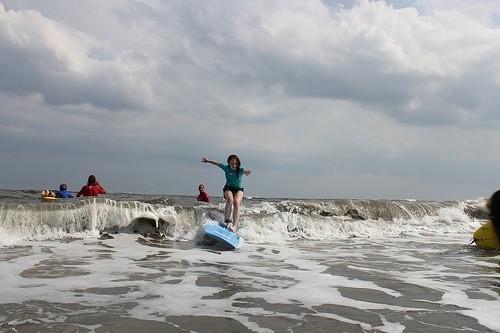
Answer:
[472,221,500,250]
[203,224,239,250]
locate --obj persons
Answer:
[197,184,211,203]
[55,184,74,199]
[487,190,500,240]
[76,175,106,197]
[201,154,253,231]
